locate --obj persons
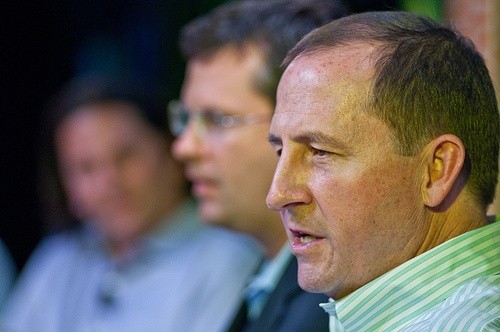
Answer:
[170,2,346,331]
[266,9,500,332]
[1,83,264,331]
